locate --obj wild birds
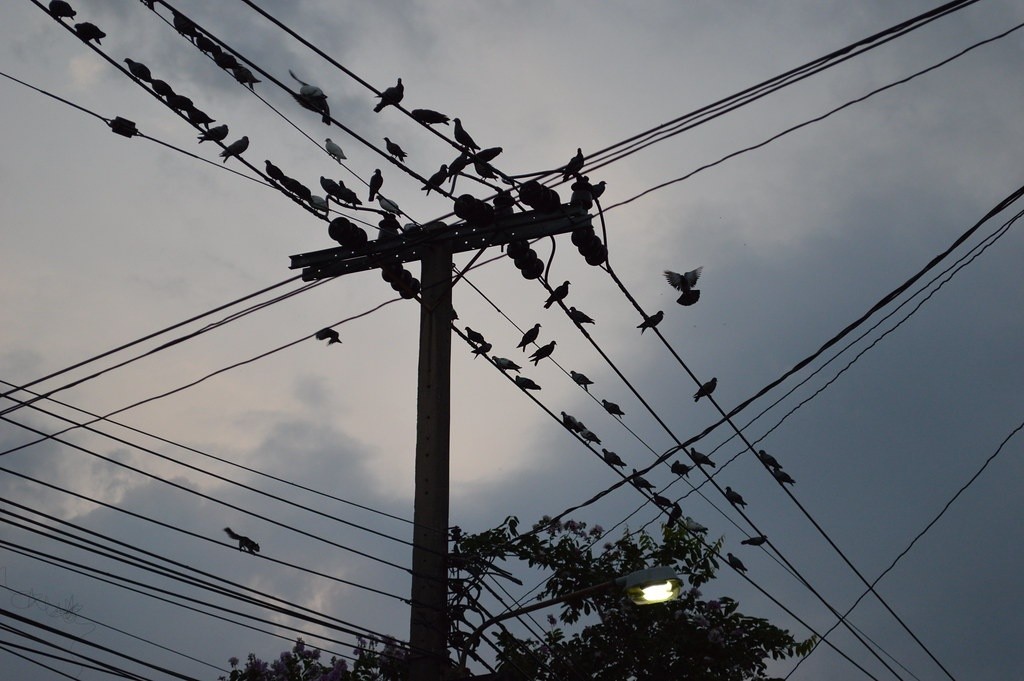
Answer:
[46,1,666,335]
[662,266,705,306]
[464,327,543,393]
[559,411,796,575]
[691,377,718,404]
[314,328,344,347]
[516,324,627,420]
[222,527,260,557]
